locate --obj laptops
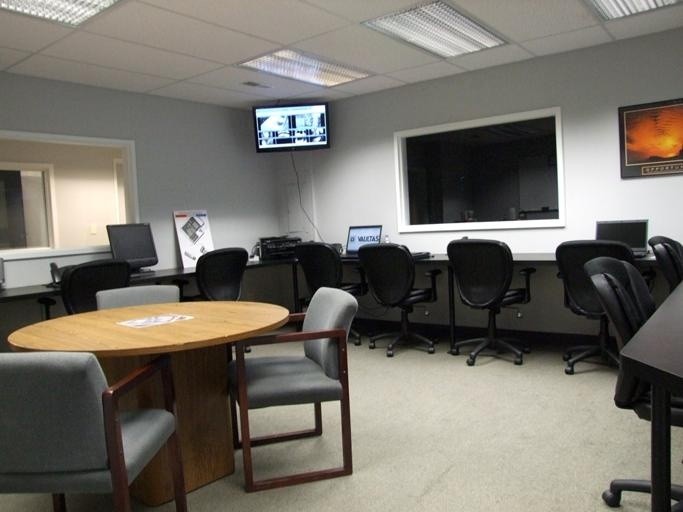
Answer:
[340,225,382,258]
[595,219,649,257]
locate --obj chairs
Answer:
[96,283,179,310]
[172,247,251,355]
[294,240,368,346]
[0,348,194,512]
[356,242,442,356]
[581,256,682,505]
[647,234,682,292]
[445,237,536,368]
[227,284,360,494]
[37,257,130,321]
[552,236,653,375]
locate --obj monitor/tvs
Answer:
[106,223,158,277]
[252,101,330,153]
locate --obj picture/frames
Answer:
[615,96,683,178]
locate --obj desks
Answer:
[1,243,683,303]
[615,280,682,512]
[7,299,292,509]
